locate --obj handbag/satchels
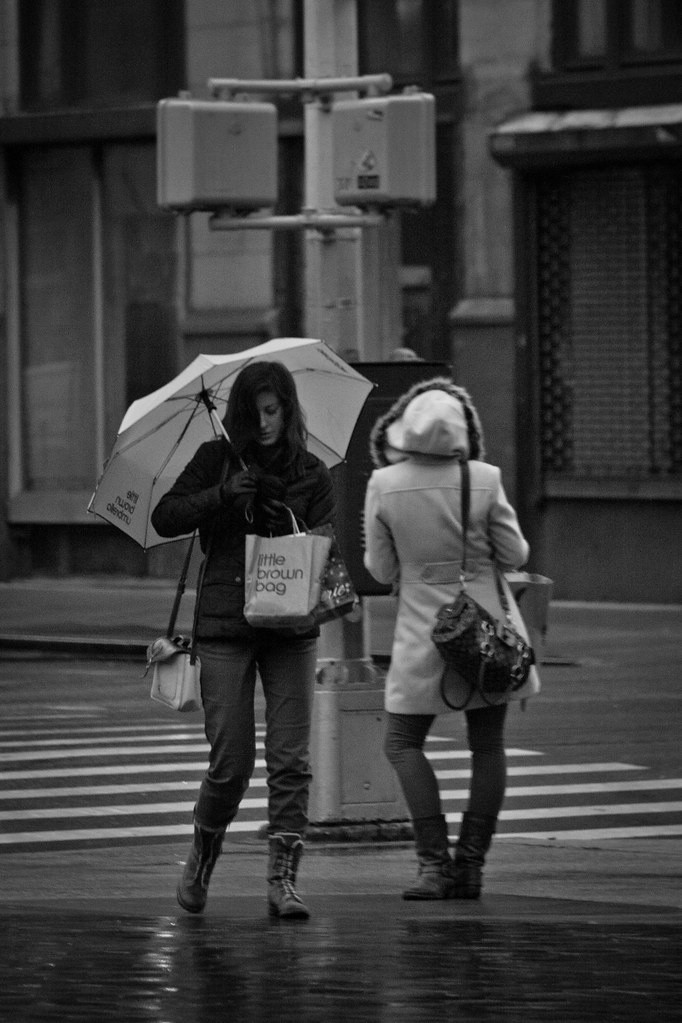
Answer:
[243,500,332,626]
[142,636,202,713]
[430,590,537,709]
[267,512,355,635]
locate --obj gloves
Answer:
[220,466,256,500]
[258,497,288,532]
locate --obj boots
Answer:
[403,815,453,900]
[176,820,230,914]
[452,810,498,901]
[265,831,310,917]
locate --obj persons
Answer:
[361,379,540,902]
[152,362,335,917]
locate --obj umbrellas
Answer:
[86,337,378,553]
